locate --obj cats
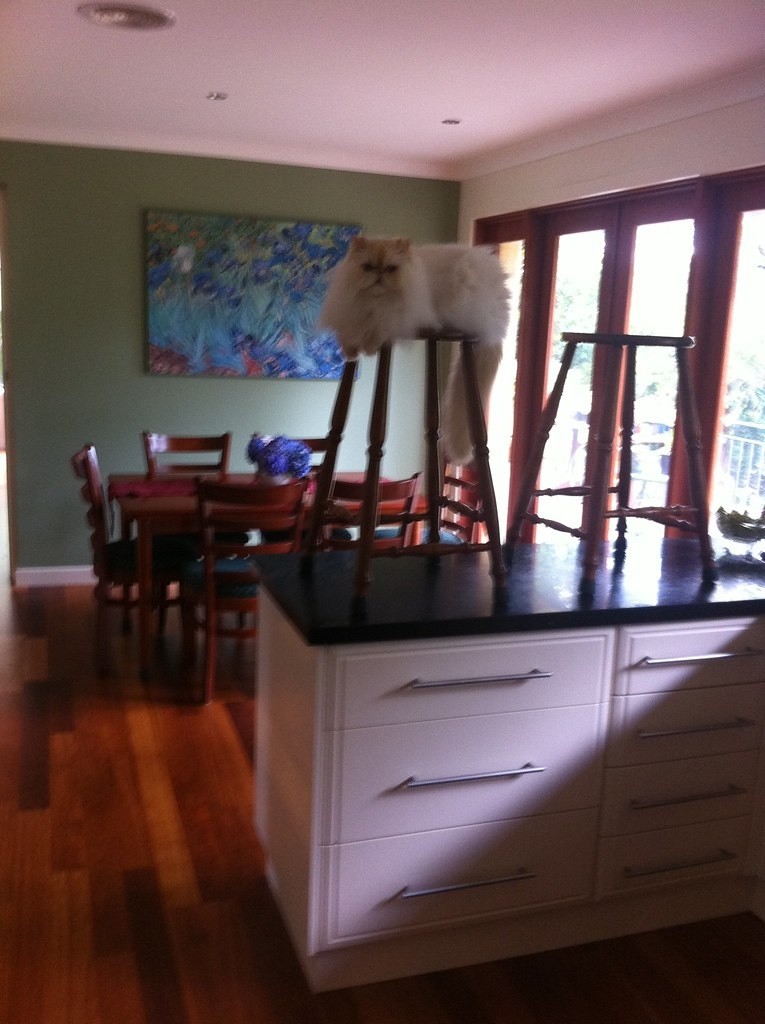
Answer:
[324,238,508,468]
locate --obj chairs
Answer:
[421,475,483,544]
[71,431,423,707]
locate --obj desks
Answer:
[106,474,432,682]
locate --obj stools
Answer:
[504,332,719,581]
[304,324,508,601]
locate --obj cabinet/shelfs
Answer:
[593,615,765,939]
[255,586,617,993]
